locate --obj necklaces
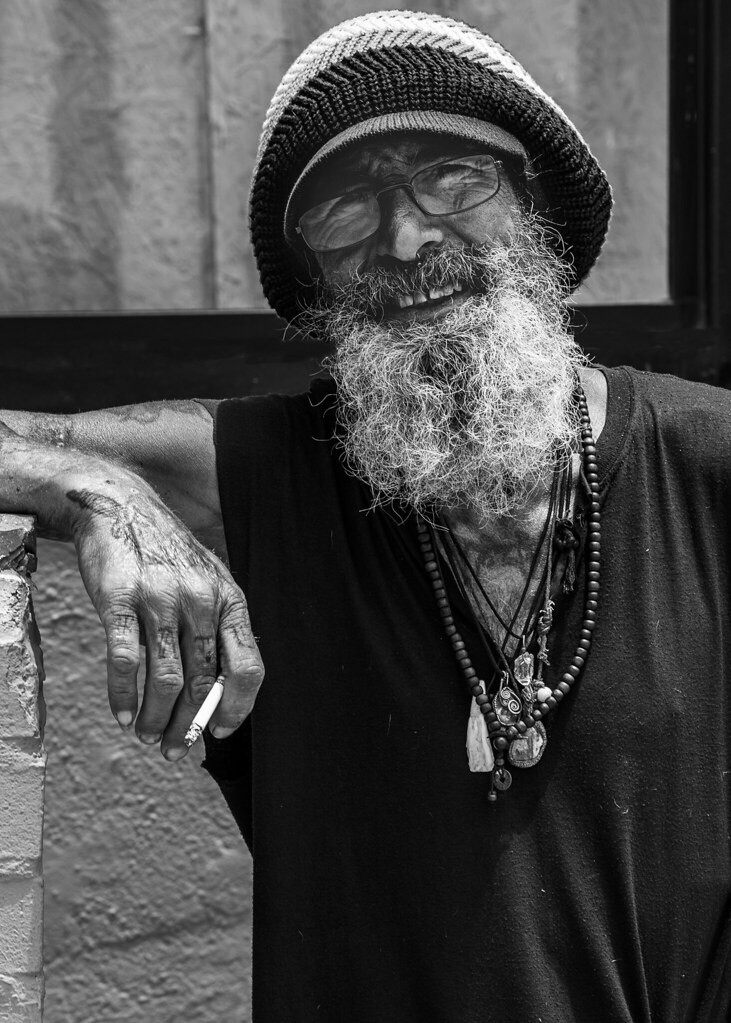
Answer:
[414,367,602,801]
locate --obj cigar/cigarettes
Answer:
[184,673,226,747]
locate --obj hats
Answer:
[249,10,615,340]
[281,109,550,254]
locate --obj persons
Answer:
[0,9,731,1023]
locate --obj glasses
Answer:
[294,155,504,255]
